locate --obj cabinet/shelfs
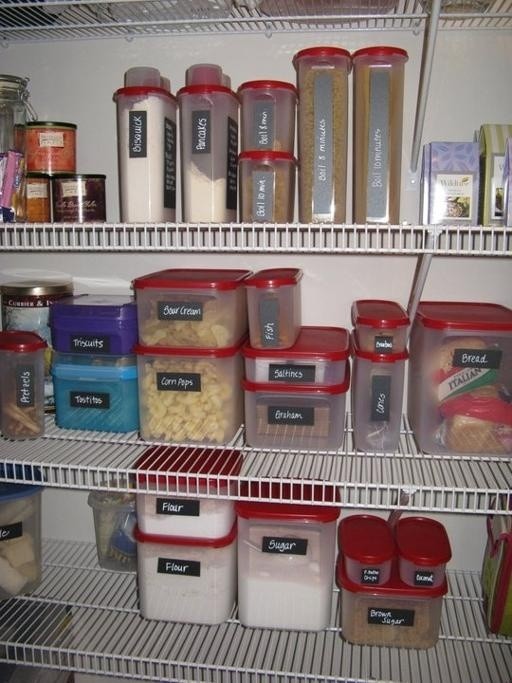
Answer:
[0,1,511,682]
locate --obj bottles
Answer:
[0,331,46,439]
[1,280,73,413]
[0,73,37,223]
